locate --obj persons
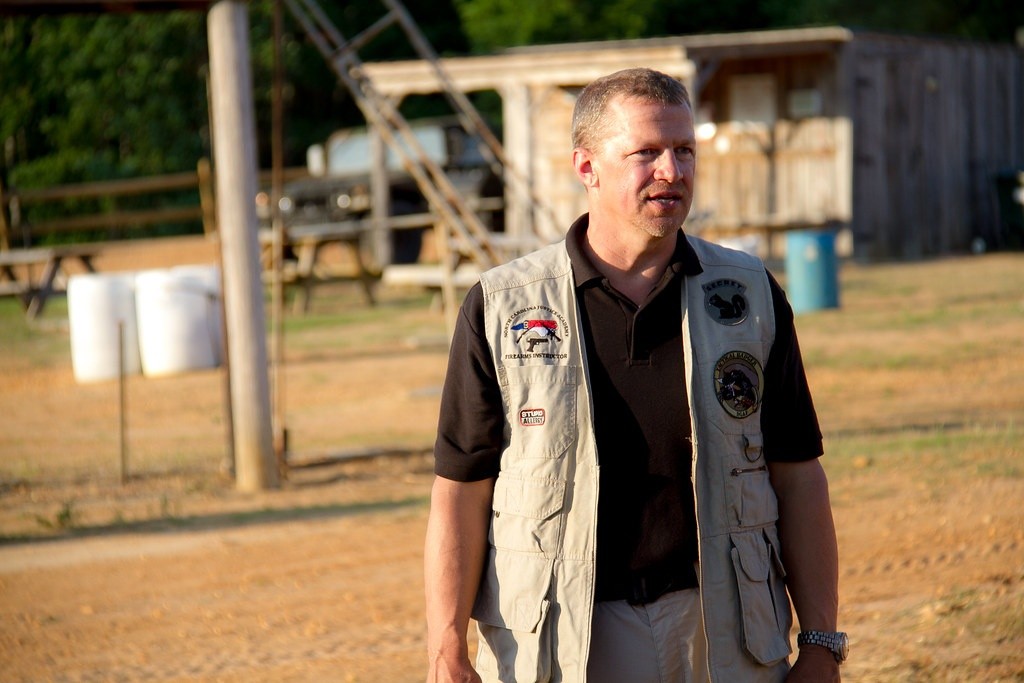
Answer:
[424,67,841,683]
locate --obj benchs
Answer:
[381,263,484,291]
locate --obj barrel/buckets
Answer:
[136,263,221,378]
[67,272,143,382]
[783,226,838,313]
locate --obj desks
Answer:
[0,244,101,320]
[259,218,384,317]
[428,234,547,315]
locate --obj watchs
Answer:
[798,631,849,664]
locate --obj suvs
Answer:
[260,116,506,264]
